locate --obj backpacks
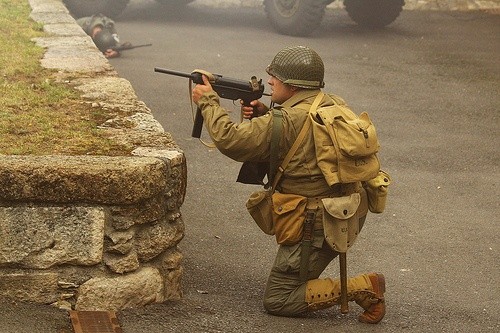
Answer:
[292,92,381,186]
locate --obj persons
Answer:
[191,45,387,326]
[74,14,120,60]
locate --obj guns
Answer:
[154,66,264,139]
[113,42,152,51]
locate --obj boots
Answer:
[305,273,387,323]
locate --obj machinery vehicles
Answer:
[65,0,406,38]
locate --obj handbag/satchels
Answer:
[246,187,276,236]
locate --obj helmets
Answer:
[265,45,325,89]
[94,28,115,54]
